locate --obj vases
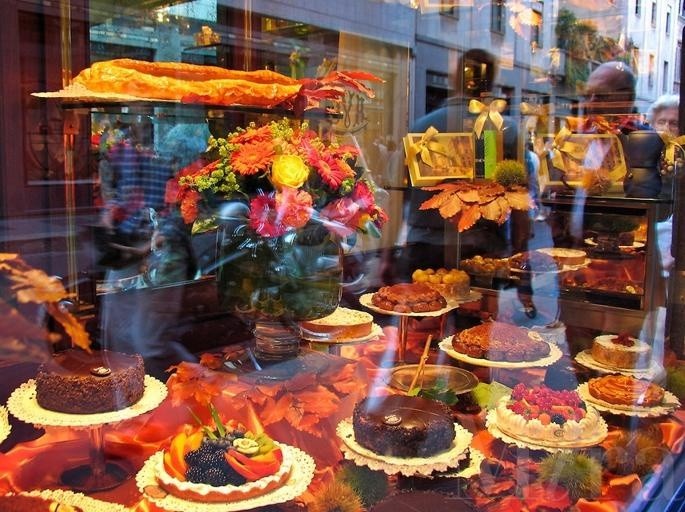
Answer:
[218,230,344,380]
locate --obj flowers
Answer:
[172,112,390,318]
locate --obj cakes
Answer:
[35,348,145,415]
[591,328,653,369]
[154,401,293,502]
[588,374,665,408]
[459,231,646,271]
[352,393,456,459]
[495,382,600,442]
[412,267,470,301]
[371,282,447,313]
[452,322,551,363]
[298,307,373,339]
